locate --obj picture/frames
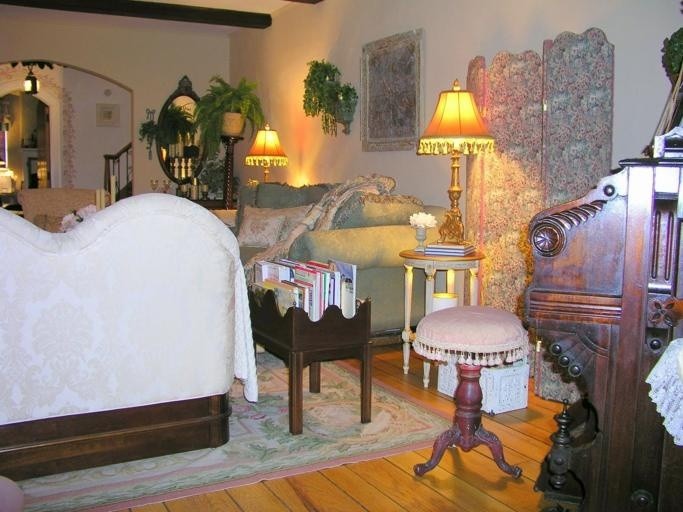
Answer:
[95,102,120,127]
[359,28,426,153]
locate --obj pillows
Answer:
[274,204,314,243]
[329,191,427,229]
[236,207,285,249]
[238,184,256,219]
[255,181,328,210]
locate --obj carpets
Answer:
[0,345,455,511]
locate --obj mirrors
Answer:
[156,74,209,184]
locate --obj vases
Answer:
[414,227,427,252]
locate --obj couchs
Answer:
[16,187,111,233]
[210,173,450,354]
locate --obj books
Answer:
[251,257,356,322]
[424,241,475,256]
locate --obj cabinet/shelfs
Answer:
[247,287,372,434]
[525,159,683,510]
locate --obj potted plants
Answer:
[190,73,264,162]
[303,59,359,137]
[138,111,163,159]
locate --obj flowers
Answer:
[409,212,437,230]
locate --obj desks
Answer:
[188,196,237,211]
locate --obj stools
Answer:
[413,305,530,479]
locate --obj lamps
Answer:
[416,78,494,256]
[23,64,40,95]
[242,123,289,184]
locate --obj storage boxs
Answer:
[437,361,529,414]
